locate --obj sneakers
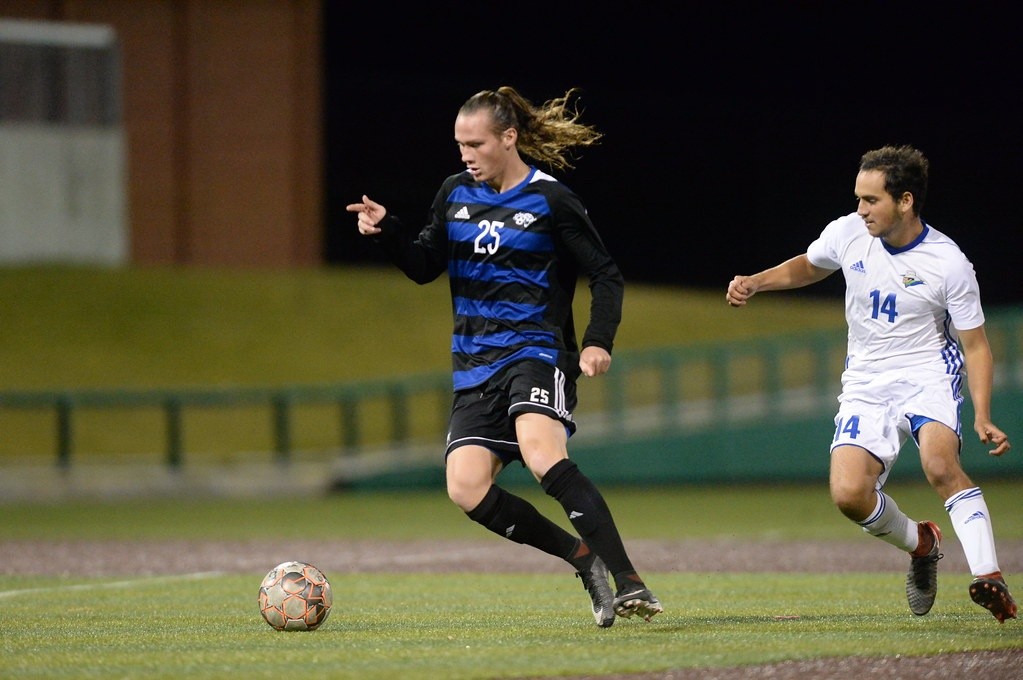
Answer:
[969,571,1017,624]
[612,580,663,623]
[574,540,616,628]
[905,520,944,616]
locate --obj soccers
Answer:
[257,560,335,634]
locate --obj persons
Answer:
[724,144,1019,624]
[347,87,665,629]
